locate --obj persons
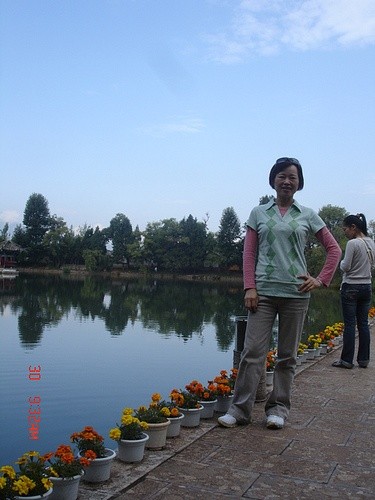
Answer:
[218,158,342,428]
[332,213,375,368]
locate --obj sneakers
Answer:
[217,414,238,427]
[266,415,284,428]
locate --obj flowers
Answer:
[109,366,238,441]
[368,307,375,319]
[0,426,108,500]
[264,323,345,371]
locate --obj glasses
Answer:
[276,157,299,167]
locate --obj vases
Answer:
[314,346,322,357]
[117,432,149,463]
[214,394,234,412]
[304,348,316,359]
[318,343,328,354]
[333,337,341,346]
[265,371,274,385]
[298,355,307,363]
[77,448,116,483]
[13,486,53,500]
[143,418,171,451]
[48,469,85,500]
[196,399,218,418]
[297,355,303,366]
[176,404,204,428]
[166,412,184,437]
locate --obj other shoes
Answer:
[332,359,350,368]
[360,365,367,368]
